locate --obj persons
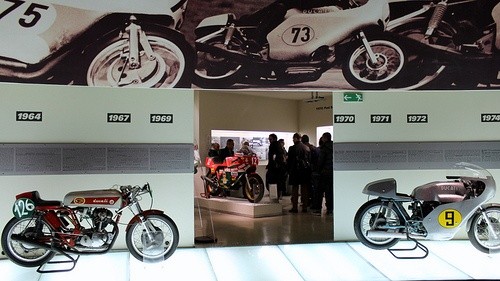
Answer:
[194,144,203,174]
[208,139,256,163]
[265,132,333,216]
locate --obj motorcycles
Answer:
[1,181,180,268]
[201,144,265,203]
[0,0,500,91]
[354,161,500,255]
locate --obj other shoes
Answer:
[311,211,322,216]
[325,211,333,216]
[289,208,298,213]
